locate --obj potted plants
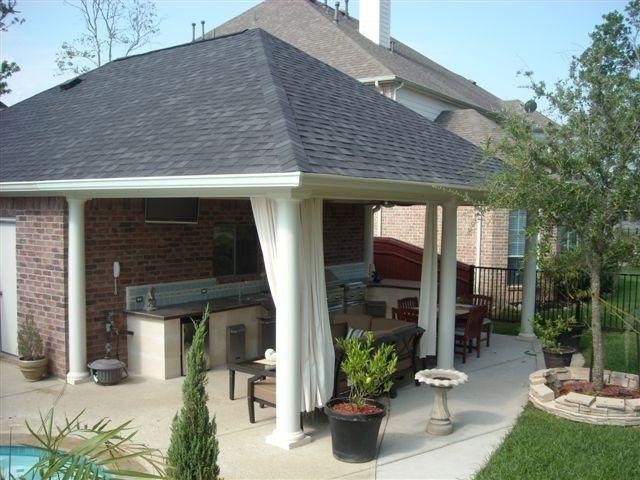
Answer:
[528,312,575,368]
[324,330,398,463]
[541,244,597,353]
[16,316,49,379]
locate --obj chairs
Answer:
[392,294,492,363]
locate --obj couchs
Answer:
[247,313,425,430]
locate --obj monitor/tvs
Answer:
[145,198,200,224]
